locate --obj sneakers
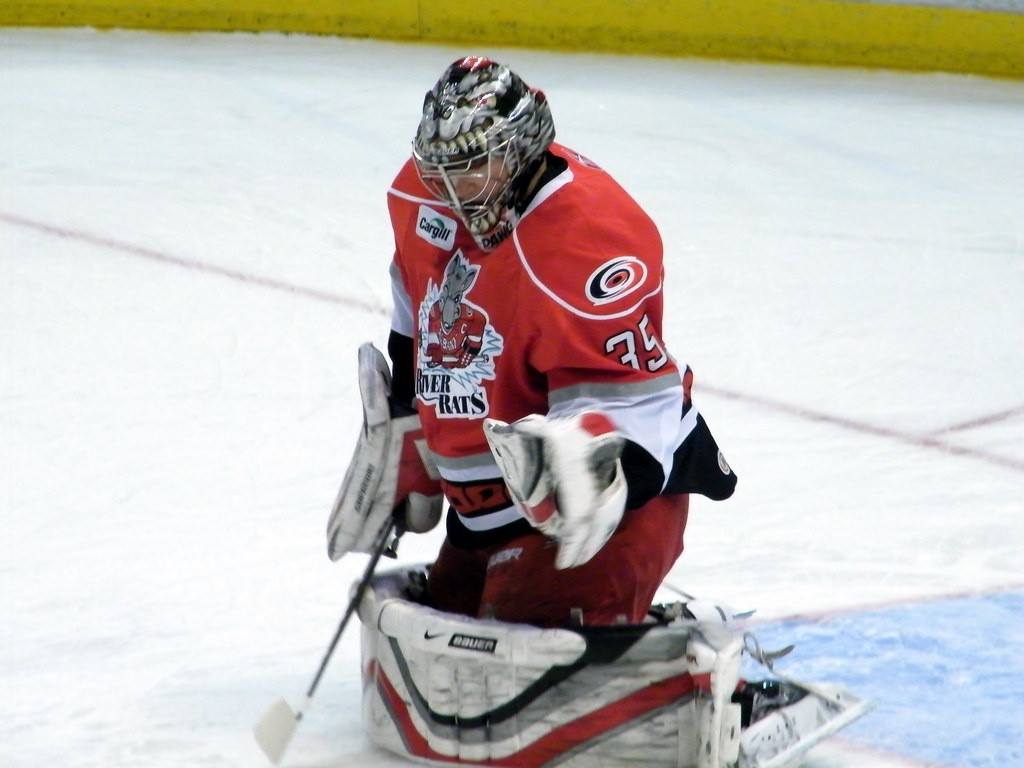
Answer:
[732,676,802,729]
[645,603,692,628]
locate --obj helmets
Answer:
[412,54,555,252]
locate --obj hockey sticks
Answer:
[250,519,398,767]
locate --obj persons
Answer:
[326,55,882,768]
